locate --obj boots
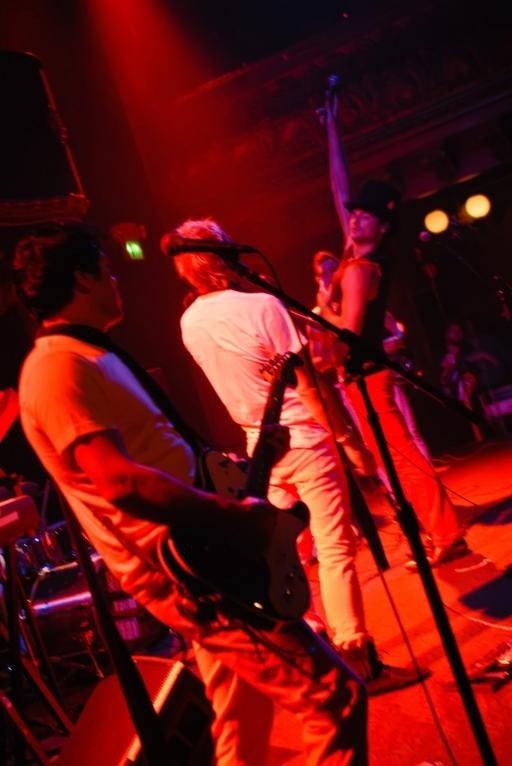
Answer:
[331,634,432,697]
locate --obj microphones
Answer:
[159,232,256,257]
[323,73,339,115]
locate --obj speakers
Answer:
[54,654,215,766]
[26,552,164,664]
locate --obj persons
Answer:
[312,91,469,574]
[10,222,368,766]
[159,222,385,681]
[433,308,512,444]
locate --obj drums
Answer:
[26,555,141,657]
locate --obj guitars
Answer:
[307,275,351,372]
[157,351,309,632]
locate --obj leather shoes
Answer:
[402,529,476,572]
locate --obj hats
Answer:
[343,178,396,227]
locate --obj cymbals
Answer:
[17,520,66,546]
